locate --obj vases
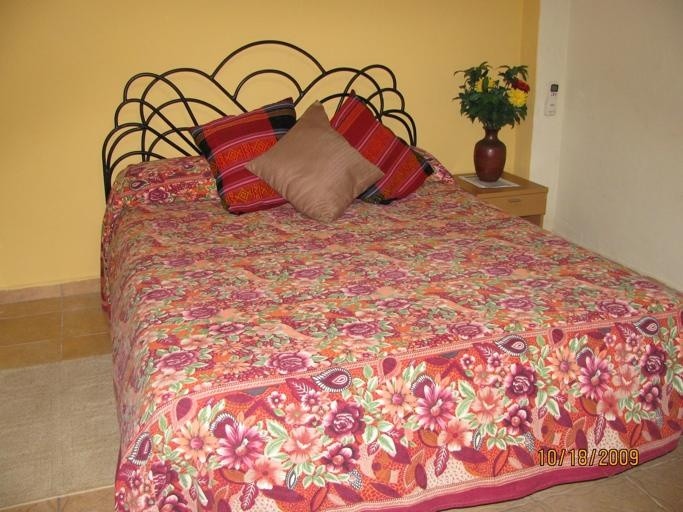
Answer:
[474,126,506,182]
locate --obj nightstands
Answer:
[452,172,548,228]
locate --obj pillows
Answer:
[329,89,433,204]
[188,96,296,213]
[244,100,385,223]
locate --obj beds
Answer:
[101,39,683,512]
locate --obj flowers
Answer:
[452,61,530,128]
